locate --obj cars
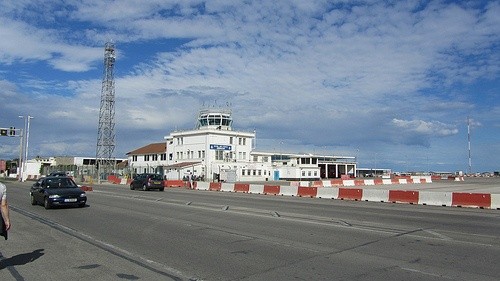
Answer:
[29,176,87,210]
[130,173,164,191]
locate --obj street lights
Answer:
[18,116,26,182]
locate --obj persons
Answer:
[0,183,12,231]
[182,175,205,181]
